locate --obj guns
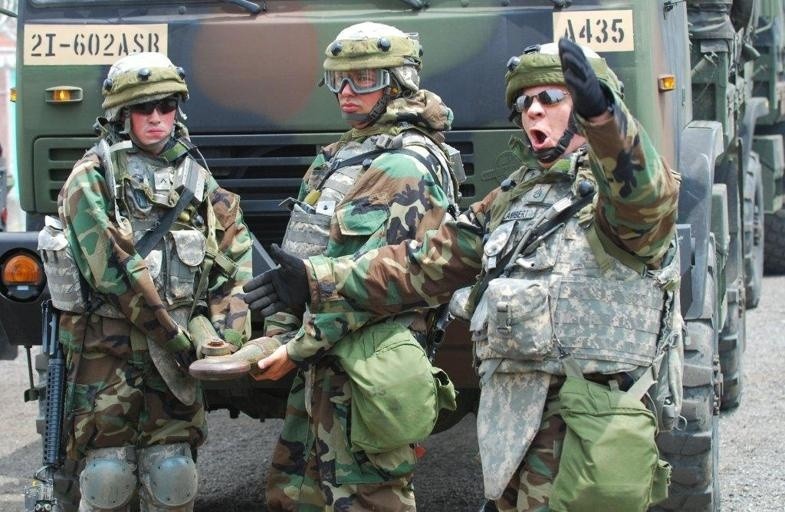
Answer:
[42,298,67,465]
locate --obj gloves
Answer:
[240,241,311,318]
[558,36,611,118]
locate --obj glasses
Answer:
[129,97,179,114]
[511,88,572,113]
[323,68,392,95]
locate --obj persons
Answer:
[52,52,253,512]
[248,21,460,512]
[243,38,682,512]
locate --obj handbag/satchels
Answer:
[326,322,461,456]
[546,375,671,512]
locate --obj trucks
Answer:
[1,0,780,512]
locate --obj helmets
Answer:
[505,39,625,130]
[321,21,425,92]
[99,51,189,111]
